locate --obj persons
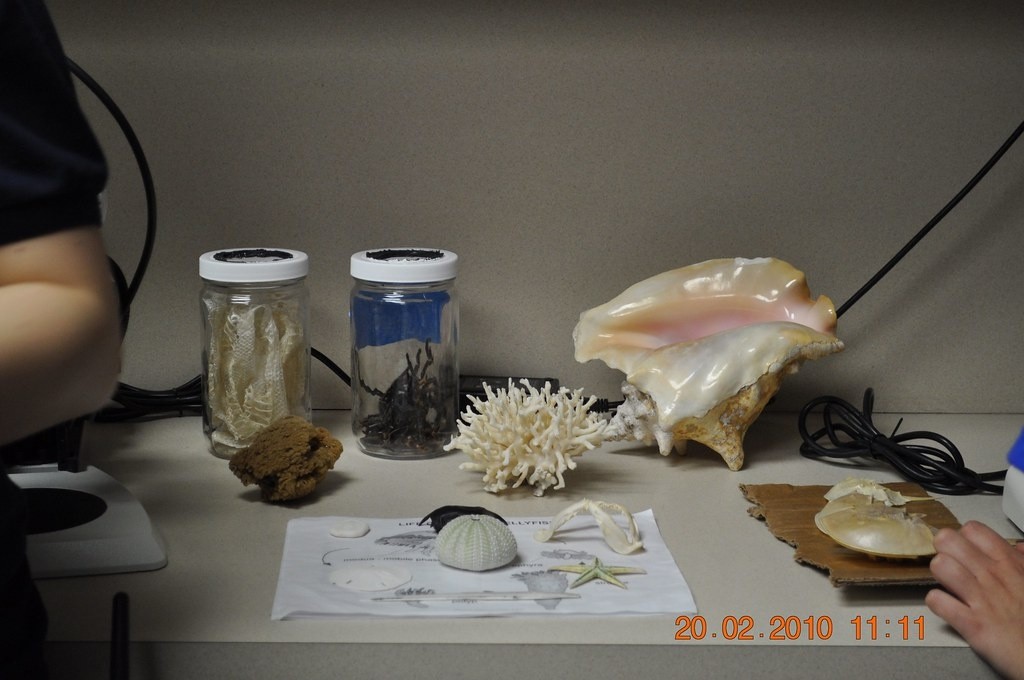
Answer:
[925,520,1024,680]
[0,1,123,680]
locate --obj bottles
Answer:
[199,247,310,459]
[350,247,460,459]
